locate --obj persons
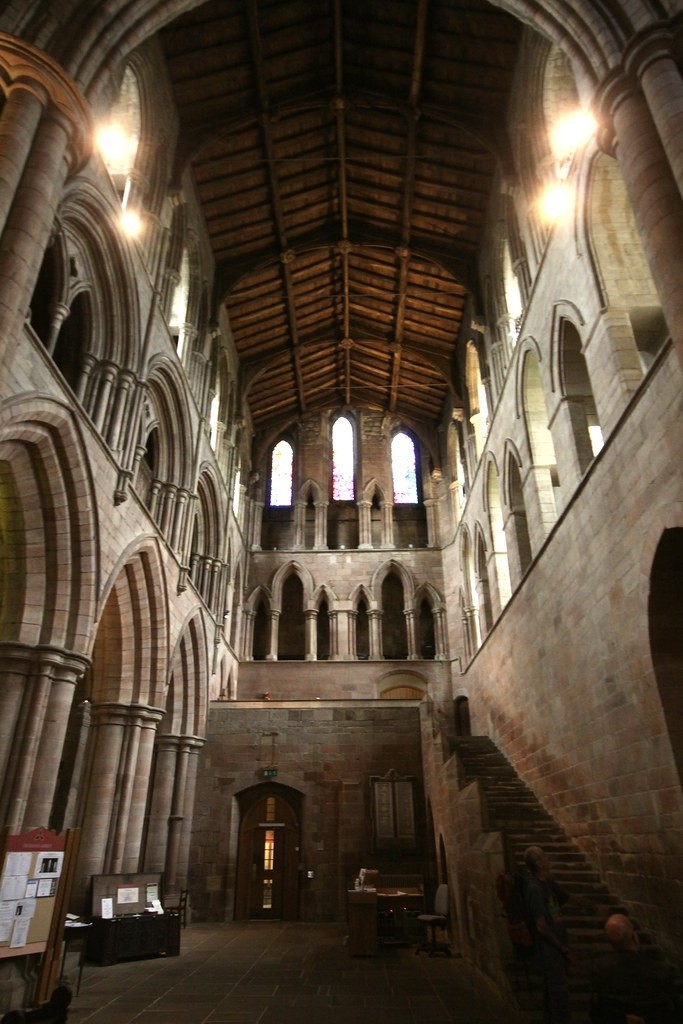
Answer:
[590,915,683,1024]
[506,846,581,1024]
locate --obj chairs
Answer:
[165,890,187,929]
[415,884,451,958]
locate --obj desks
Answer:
[59,926,93,997]
[86,910,181,966]
[376,883,426,931]
